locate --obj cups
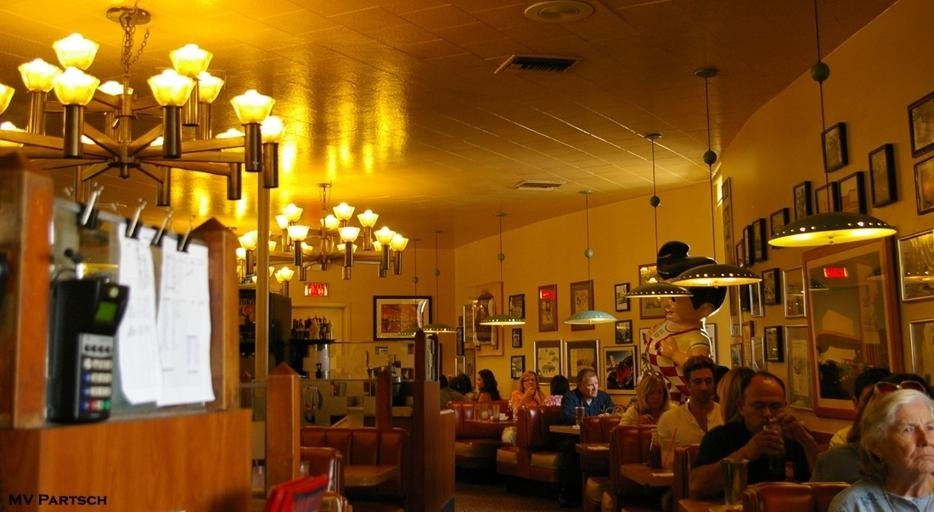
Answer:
[721,457,751,512]
[479,402,488,420]
[575,406,585,427]
[763,425,786,457]
[492,404,501,422]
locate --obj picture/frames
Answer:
[454,263,718,396]
[291,296,437,408]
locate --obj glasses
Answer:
[874,380,925,398]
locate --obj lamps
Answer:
[235,239,295,291]
[625,134,694,298]
[478,210,526,325]
[670,67,763,288]
[421,229,458,333]
[768,1,898,248]
[239,183,408,281]
[0,6,284,206]
[563,188,617,325]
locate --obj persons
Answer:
[619,371,679,427]
[717,367,756,422]
[689,372,822,500]
[829,368,892,449]
[392,383,413,406]
[502,371,546,446]
[828,389,934,512]
[440,374,471,410]
[811,373,934,483]
[650,356,726,468]
[557,370,615,508]
[543,375,570,407]
[447,374,472,395]
[476,369,500,400]
[645,241,727,407]
[712,366,729,403]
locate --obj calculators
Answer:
[56,277,130,424]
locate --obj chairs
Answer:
[263,426,412,512]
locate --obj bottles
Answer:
[648,429,662,472]
[292,314,332,342]
